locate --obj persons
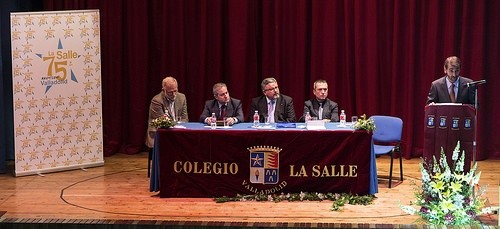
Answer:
[425,56,475,105]
[199,83,244,126]
[303,79,339,122]
[146,76,189,147]
[247,77,296,123]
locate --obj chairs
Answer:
[369,115,403,188]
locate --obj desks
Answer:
[149,121,380,198]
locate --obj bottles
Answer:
[305,112,311,128]
[165,110,169,117]
[210,113,216,130]
[339,109,347,128]
[253,110,260,128]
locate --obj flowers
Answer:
[403,140,500,229]
[213,191,374,211]
[352,114,377,134]
[148,114,178,129]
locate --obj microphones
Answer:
[463,80,486,88]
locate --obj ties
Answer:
[449,84,455,103]
[269,101,273,114]
[319,102,323,120]
[220,105,227,120]
[171,101,175,122]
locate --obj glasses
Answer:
[264,87,278,92]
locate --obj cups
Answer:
[223,117,229,128]
[352,116,358,126]
[264,117,271,127]
